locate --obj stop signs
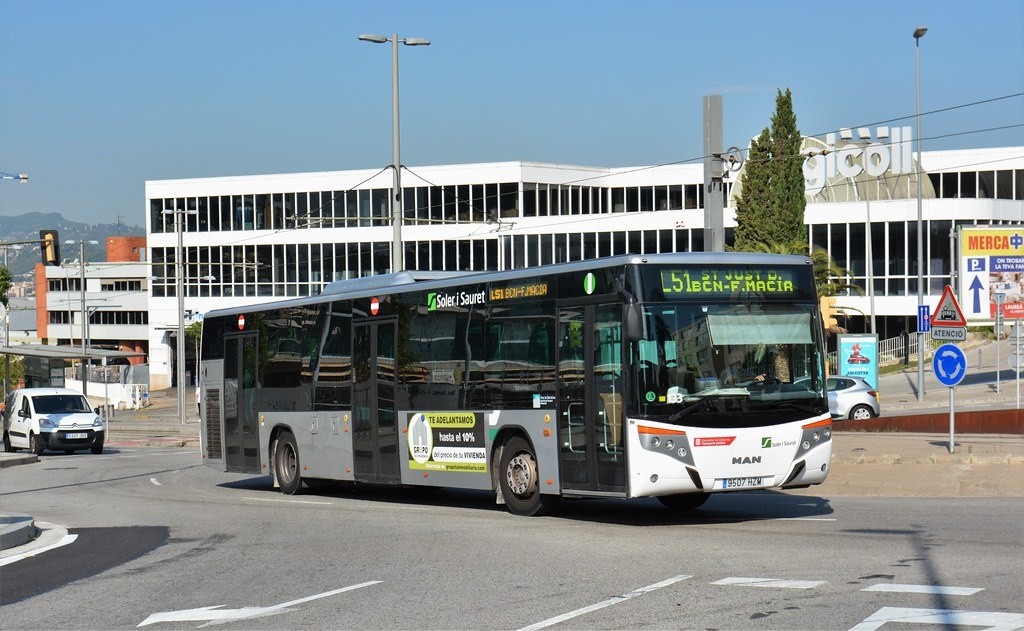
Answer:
[370,296,379,315]
[237,315,245,330]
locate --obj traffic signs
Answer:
[917,305,932,334]
[954,223,1024,326]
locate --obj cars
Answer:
[792,374,881,421]
[848,354,870,365]
[2,388,106,456]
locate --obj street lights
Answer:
[356,29,432,272]
[161,207,215,424]
[65,239,100,397]
[913,25,929,400]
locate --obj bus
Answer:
[200,253,832,516]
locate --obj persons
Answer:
[196,386,201,422]
[720,348,767,383]
